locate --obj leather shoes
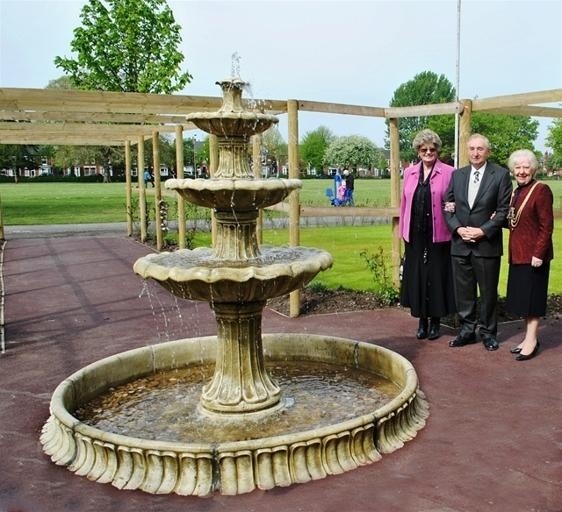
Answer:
[482,336,499,351]
[515,340,541,361]
[512,347,522,353]
[448,337,476,347]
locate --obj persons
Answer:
[344,169,354,204]
[398,129,457,340]
[489,149,556,362]
[338,180,347,201]
[442,133,512,352]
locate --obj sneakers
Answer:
[416,319,429,339]
[429,321,441,340]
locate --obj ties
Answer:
[474,171,479,183]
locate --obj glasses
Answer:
[419,148,437,152]
